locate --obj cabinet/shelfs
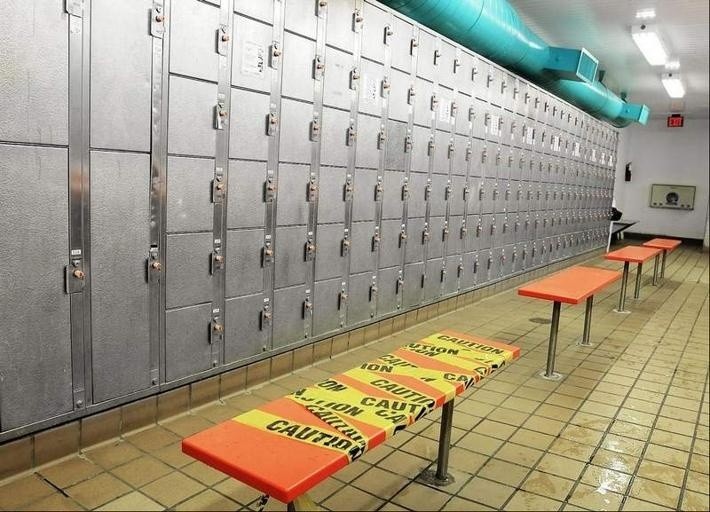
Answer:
[312,0,363,341]
[271,1,324,356]
[344,0,619,330]
[0,0,85,442]
[164,0,229,390]
[84,0,162,415]
[222,0,282,371]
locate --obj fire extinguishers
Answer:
[625,161,632,181]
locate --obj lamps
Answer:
[631,24,686,99]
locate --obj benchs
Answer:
[604,245,661,312]
[518,265,623,379]
[181,327,520,512]
[643,239,681,285]
